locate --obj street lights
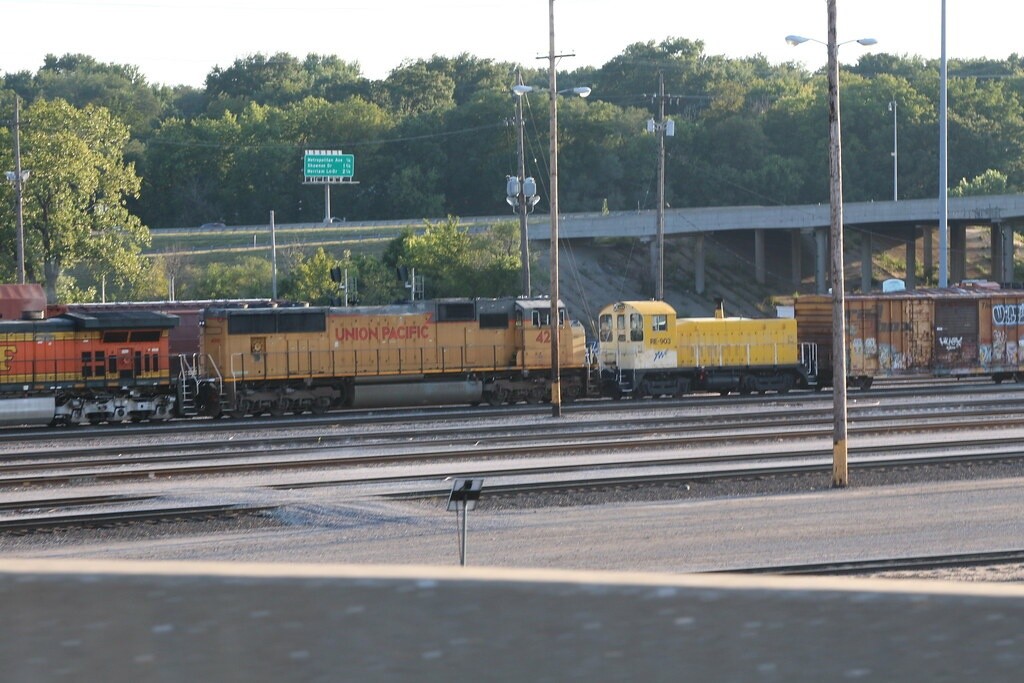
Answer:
[784,34,878,490]
[514,84,592,417]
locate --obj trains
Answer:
[0,280,1024,432]
